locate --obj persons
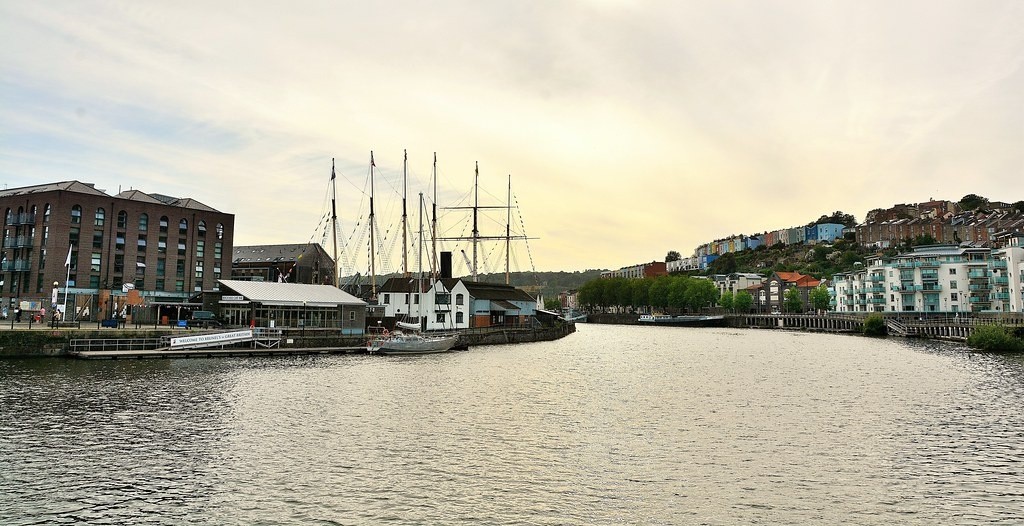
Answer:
[40,306,45,323]
[15,305,22,323]
[54,309,61,321]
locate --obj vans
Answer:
[186,310,220,325]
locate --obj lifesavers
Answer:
[382,329,389,337]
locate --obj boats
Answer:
[366,331,461,354]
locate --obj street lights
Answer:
[959,290,963,323]
[944,296,947,324]
[963,292,967,322]
[896,297,899,323]
[1020,287,1024,323]
[996,285,1000,324]
[918,298,921,322]
